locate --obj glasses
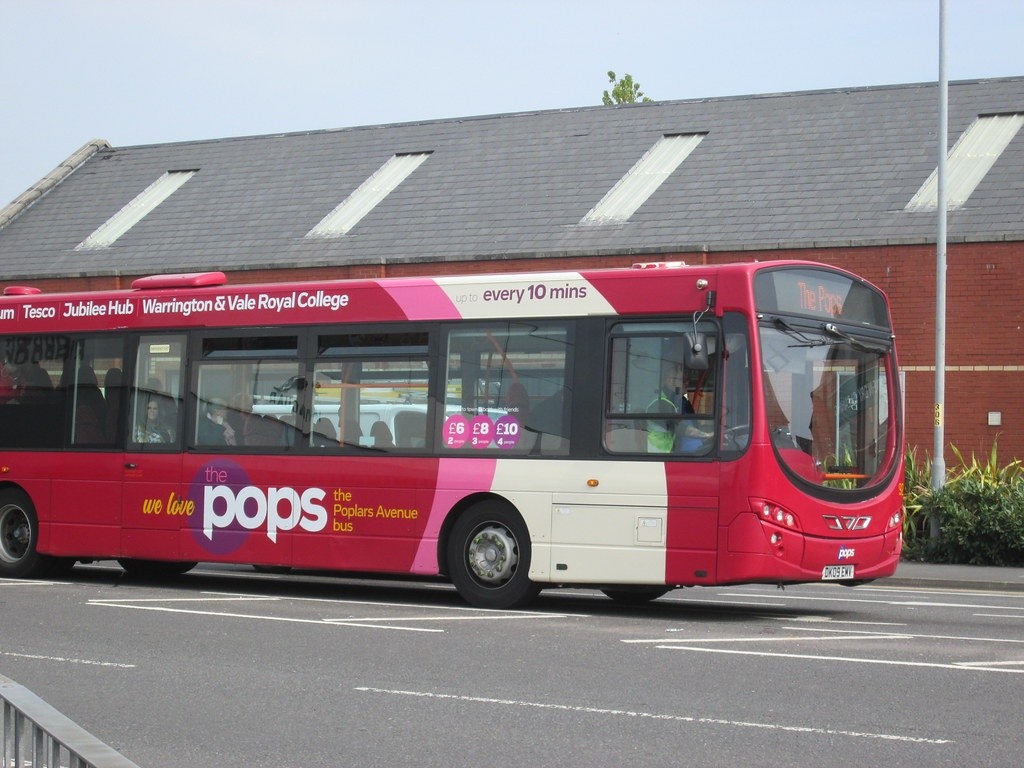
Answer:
[668,377,687,383]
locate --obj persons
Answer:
[0,352,34,406]
[646,366,714,454]
[504,383,530,418]
[133,377,254,446]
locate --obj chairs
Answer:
[18,366,649,454]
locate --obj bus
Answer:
[1,257,907,609]
